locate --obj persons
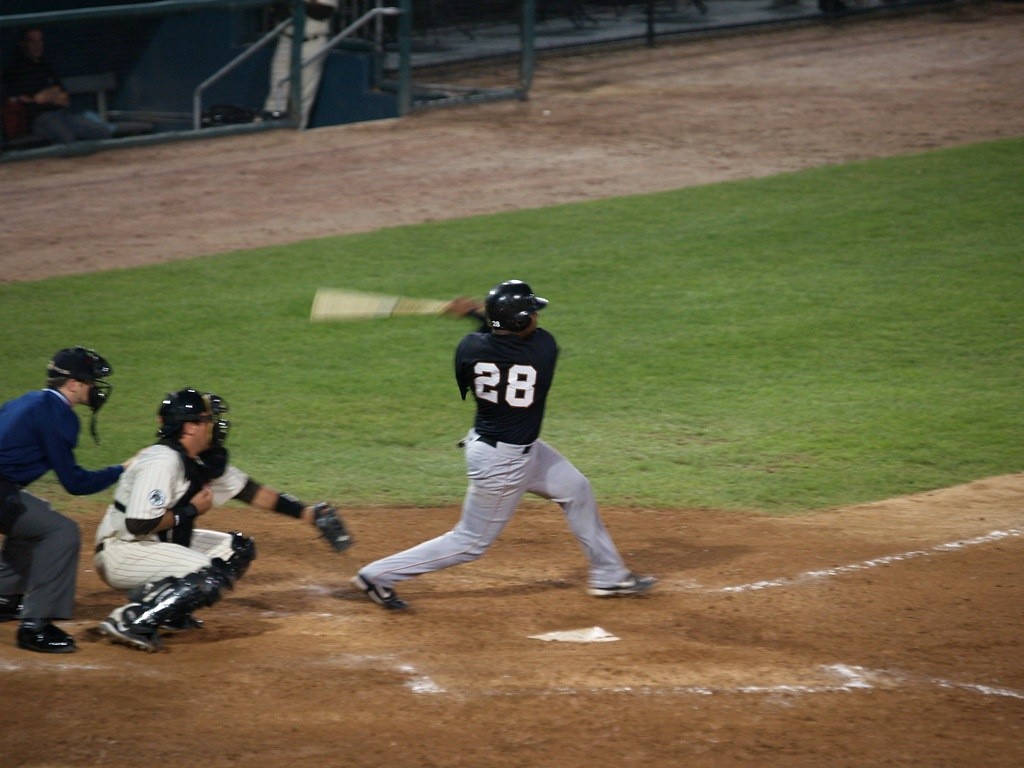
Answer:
[349,278,657,611]
[89,386,357,655]
[14,26,118,141]
[0,345,138,655]
[249,0,341,131]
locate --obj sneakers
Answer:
[0,595,26,620]
[586,573,655,596]
[98,608,158,653]
[159,611,195,631]
[350,574,409,609]
[16,620,74,653]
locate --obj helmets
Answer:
[158,388,210,418]
[47,348,112,384]
[485,280,549,332]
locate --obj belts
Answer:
[96,542,104,554]
[477,436,532,454]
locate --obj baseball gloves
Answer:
[312,500,360,553]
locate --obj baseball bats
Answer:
[309,286,453,325]
